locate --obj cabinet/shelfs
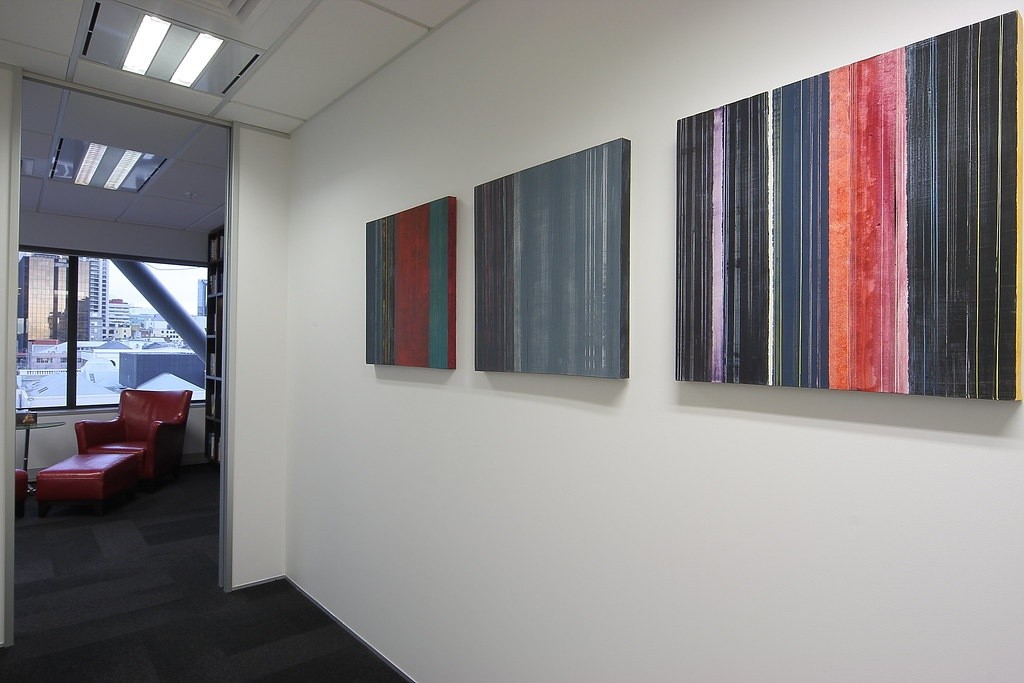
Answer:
[204,223,225,465]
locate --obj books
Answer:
[208,433,215,458]
[212,235,225,260]
[210,353,215,377]
[210,393,215,416]
[218,436,223,461]
[210,274,223,293]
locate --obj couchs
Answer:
[74,389,193,496]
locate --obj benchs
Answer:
[36,454,138,518]
